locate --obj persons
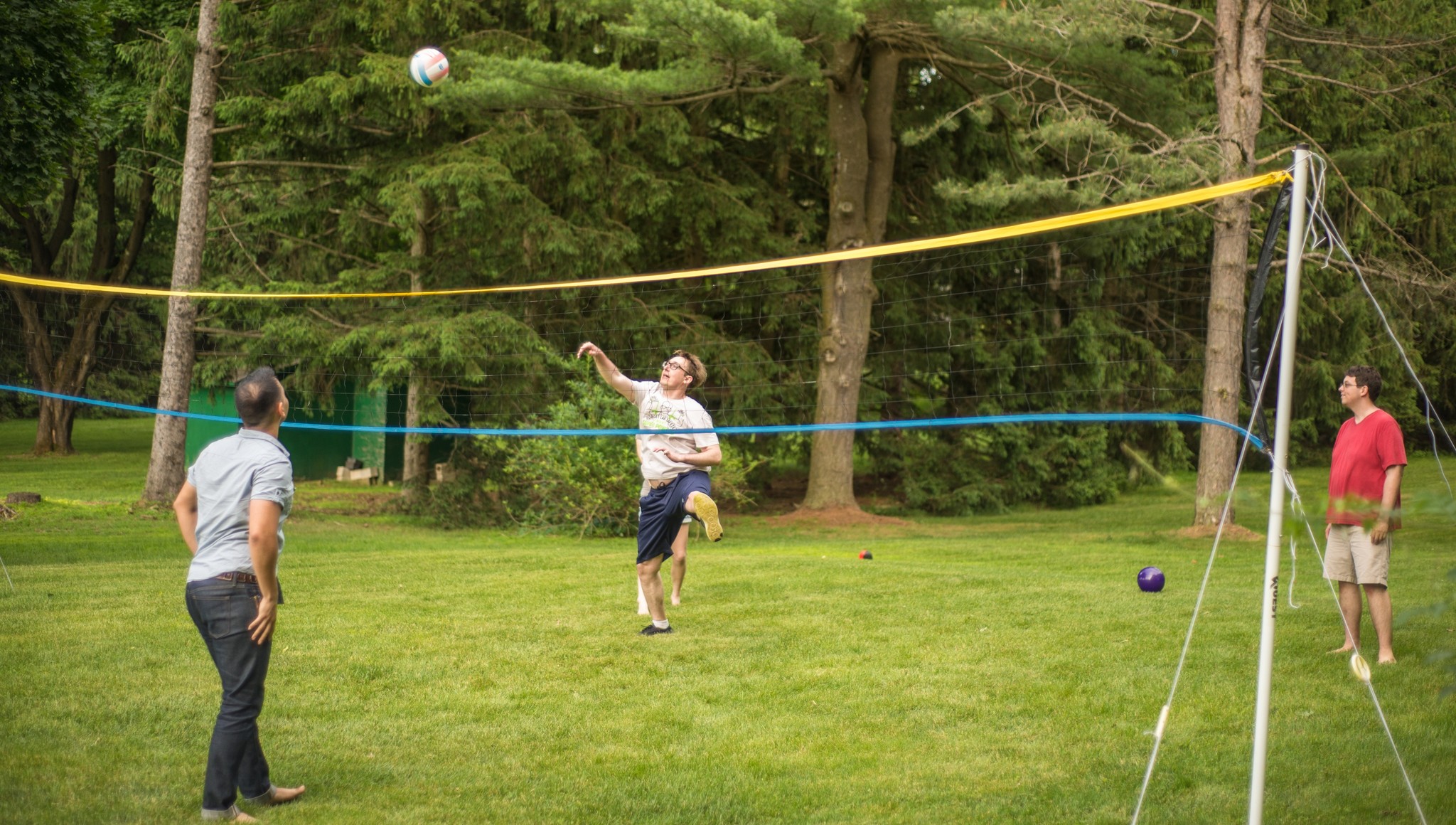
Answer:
[172,365,306,824]
[575,340,723,638]
[1322,368,1408,668]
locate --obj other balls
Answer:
[859,550,873,559]
[1137,567,1165,592]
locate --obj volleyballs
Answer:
[409,48,449,88]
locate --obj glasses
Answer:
[662,361,692,376]
[1340,383,1363,388]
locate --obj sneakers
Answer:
[692,492,723,542]
[637,623,673,636]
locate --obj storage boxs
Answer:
[336,458,378,485]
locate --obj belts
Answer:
[215,572,259,584]
